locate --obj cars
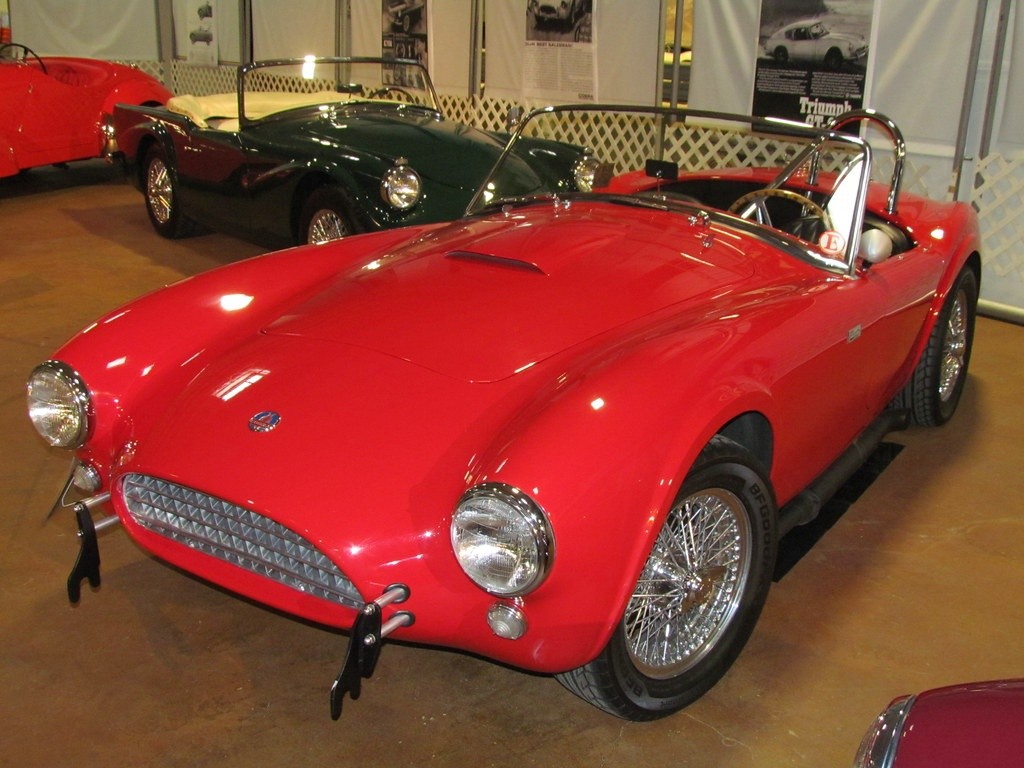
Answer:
[762,21,867,70]
[188,27,214,46]
[534,0,586,28]
[388,1,426,32]
[196,1,213,20]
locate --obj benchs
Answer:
[26,58,113,86]
[682,179,910,254]
[166,90,357,128]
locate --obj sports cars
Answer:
[0,42,178,177]
[24,102,982,723]
[98,54,600,246]
